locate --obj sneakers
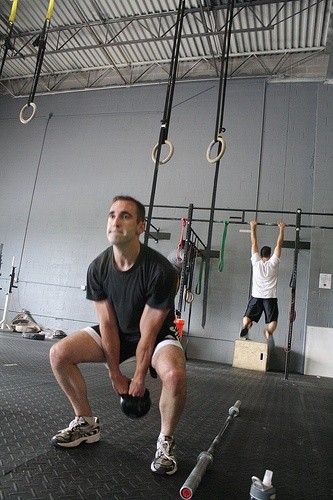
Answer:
[50,416,103,448]
[150,432,178,475]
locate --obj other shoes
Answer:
[240,322,253,337]
[264,330,273,340]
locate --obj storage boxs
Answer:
[232,340,269,371]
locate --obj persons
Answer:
[48,195,187,475]
[239,220,285,351]
